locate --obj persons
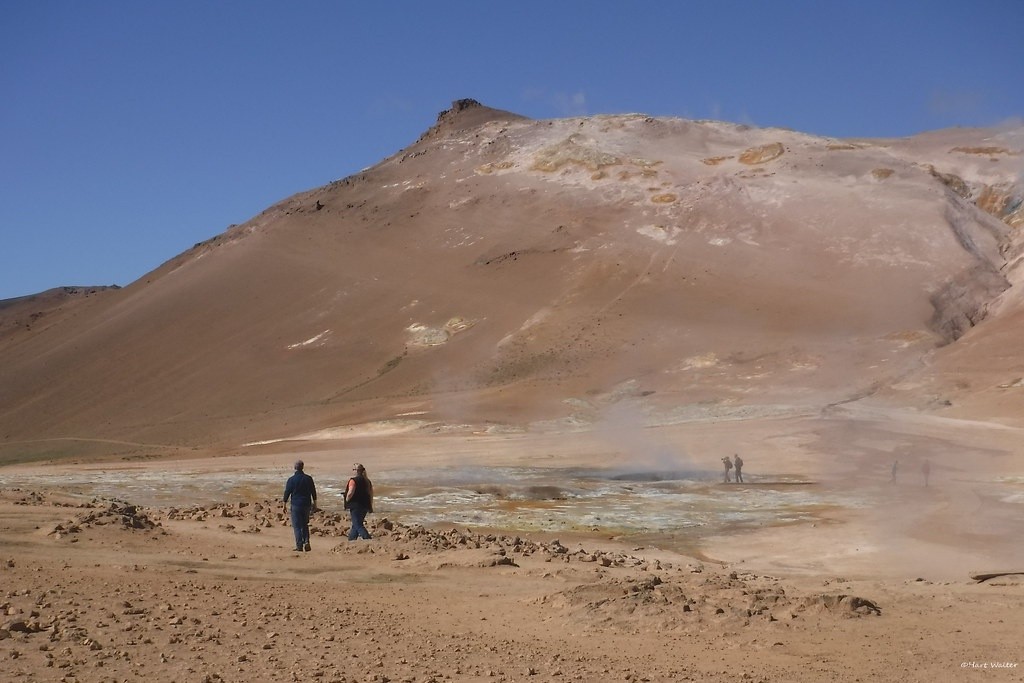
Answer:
[344,463,374,541]
[723,457,731,482]
[733,454,743,483]
[283,460,317,551]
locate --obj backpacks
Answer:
[728,461,733,469]
[738,459,743,467]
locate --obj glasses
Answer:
[353,469,356,471]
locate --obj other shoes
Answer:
[305,543,311,551]
[293,547,303,551]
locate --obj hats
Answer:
[294,459,304,469]
